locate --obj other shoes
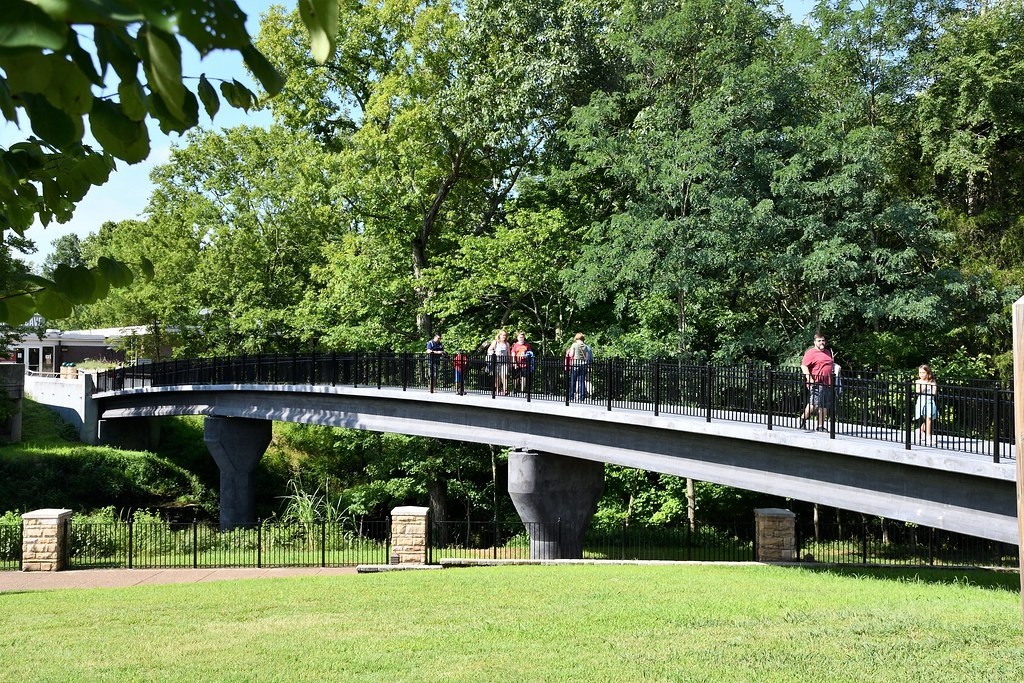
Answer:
[926,436,936,445]
[915,428,926,441]
[816,426,828,432]
[800,418,807,430]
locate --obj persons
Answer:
[914,364,939,434]
[795,333,834,433]
[426,329,531,395]
[569,332,588,401]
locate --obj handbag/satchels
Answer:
[486,354,497,366]
[587,363,593,373]
